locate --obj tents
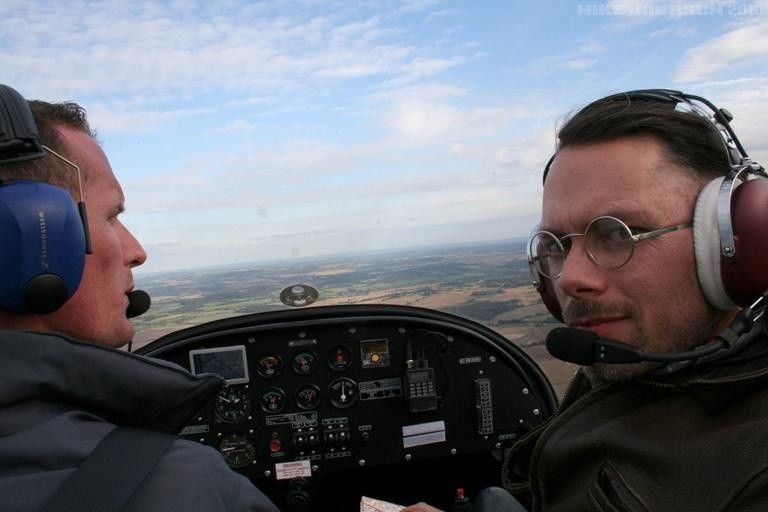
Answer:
[538,90,768,310]
[0,84,92,315]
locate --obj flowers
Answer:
[546,307,761,367]
[126,290,152,319]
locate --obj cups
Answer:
[529,212,693,280]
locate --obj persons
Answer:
[394,86,766,512]
[0,82,288,512]
